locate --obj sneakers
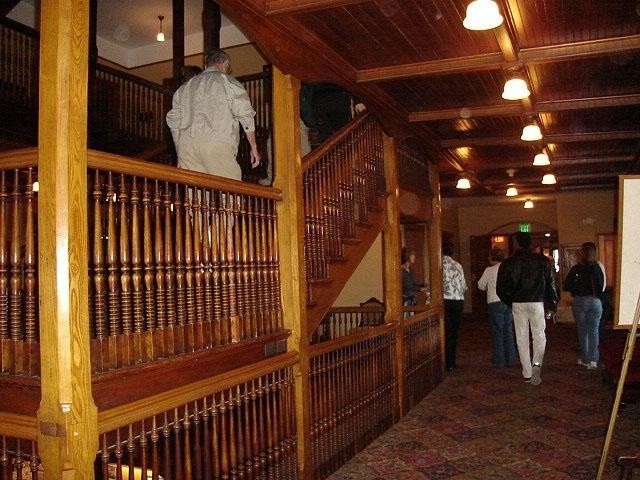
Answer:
[524,362,540,385]
[577,359,597,370]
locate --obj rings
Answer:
[257,161,260,165]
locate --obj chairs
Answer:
[352,296,386,356]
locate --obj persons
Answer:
[398,229,608,387]
[258,80,313,188]
[166,49,262,247]
[165,65,204,214]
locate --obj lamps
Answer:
[155,13,167,43]
[518,119,544,143]
[499,73,531,103]
[530,151,552,167]
[462,0,505,33]
[503,182,520,199]
[453,178,471,190]
[523,196,534,209]
[539,168,560,189]
